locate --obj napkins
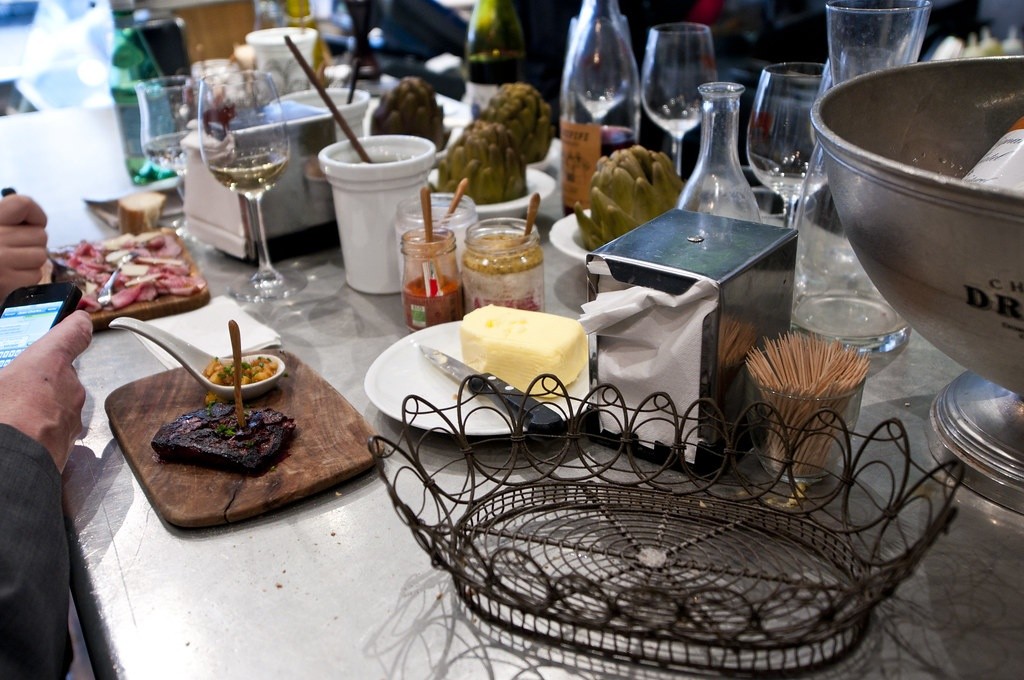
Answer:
[585,255,726,465]
[128,294,281,369]
[180,117,252,262]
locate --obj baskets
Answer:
[367,374,968,680]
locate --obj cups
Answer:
[270,86,371,145]
[316,134,437,296]
[740,164,818,230]
[244,26,320,97]
[741,349,867,488]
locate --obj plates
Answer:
[365,320,596,436]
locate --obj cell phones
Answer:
[0,281,83,370]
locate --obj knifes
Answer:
[420,344,565,438]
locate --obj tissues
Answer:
[579,205,797,475]
[185,98,338,267]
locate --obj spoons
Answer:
[108,316,286,402]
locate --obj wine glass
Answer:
[641,21,718,177]
[196,69,308,303]
[746,61,827,227]
[132,73,193,241]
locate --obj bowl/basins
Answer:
[527,136,562,170]
[429,168,559,221]
[548,208,592,265]
[362,118,465,164]
[810,55,1024,519]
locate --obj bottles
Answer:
[464,0,526,120]
[395,190,546,332]
[106,0,180,186]
[560,0,642,216]
[792,0,934,358]
[673,81,762,225]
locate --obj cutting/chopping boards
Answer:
[49,226,210,334]
[104,348,386,530]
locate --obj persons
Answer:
[0,194,93,680]
[493,0,983,179]
